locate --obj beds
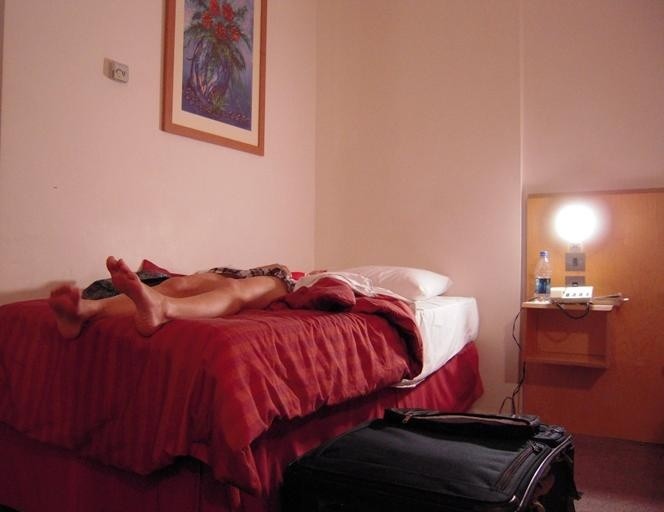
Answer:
[1,264,483,511]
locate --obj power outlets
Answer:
[564,274,586,286]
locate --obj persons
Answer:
[46,255,329,342]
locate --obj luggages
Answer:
[276,401,583,512]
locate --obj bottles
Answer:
[534,251,551,303]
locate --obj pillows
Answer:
[337,267,452,302]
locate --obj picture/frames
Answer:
[160,1,272,160]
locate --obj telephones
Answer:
[549,285,594,303]
